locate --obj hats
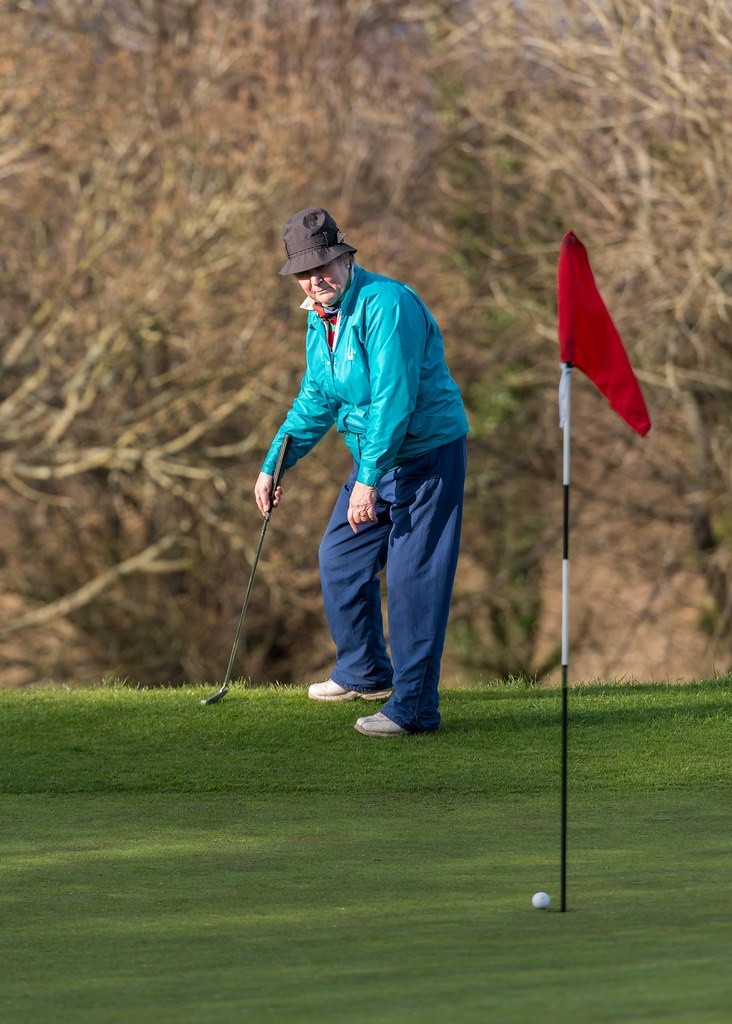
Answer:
[278,207,358,276]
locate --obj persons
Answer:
[254,207,470,737]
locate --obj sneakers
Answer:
[309,677,393,701]
[355,709,442,737]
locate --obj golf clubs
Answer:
[199,431,294,708]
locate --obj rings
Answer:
[360,517,366,521]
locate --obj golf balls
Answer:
[531,891,550,910]
